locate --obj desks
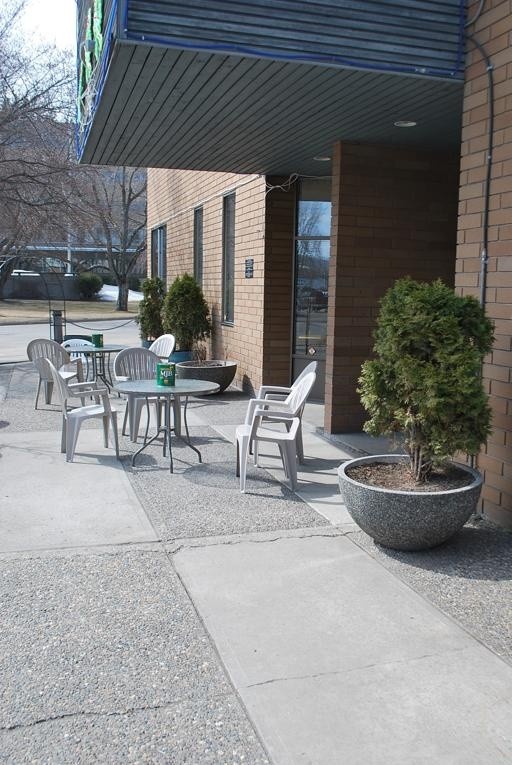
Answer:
[114,380,221,474]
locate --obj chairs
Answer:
[235,370,317,495]
[246,360,317,467]
[27,332,180,462]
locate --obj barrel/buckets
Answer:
[157,364,176,387]
[92,333,104,348]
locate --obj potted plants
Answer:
[158,269,217,375]
[334,275,498,552]
[133,275,168,350]
